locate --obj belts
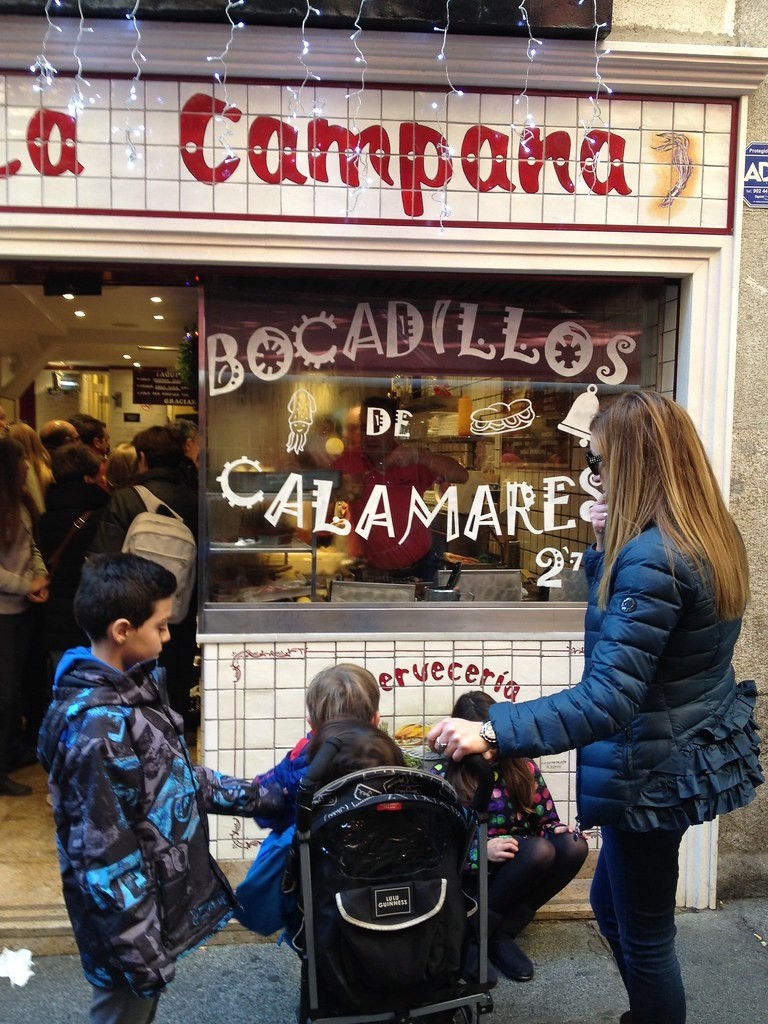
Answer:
[365,548,437,578]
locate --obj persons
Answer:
[0,412,203,796]
[427,384,766,1024]
[231,660,591,992]
[36,550,291,1024]
[329,396,469,572]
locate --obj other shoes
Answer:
[5,746,37,773]
[0,773,33,796]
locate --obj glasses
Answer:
[584,450,607,477]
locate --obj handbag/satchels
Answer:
[222,817,302,932]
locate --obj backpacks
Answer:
[118,484,197,625]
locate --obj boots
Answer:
[489,901,536,981]
[463,897,498,990]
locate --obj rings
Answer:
[436,737,447,749]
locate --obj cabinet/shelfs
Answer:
[205,492,318,602]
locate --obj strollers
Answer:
[278,736,500,1024]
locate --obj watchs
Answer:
[479,719,500,751]
